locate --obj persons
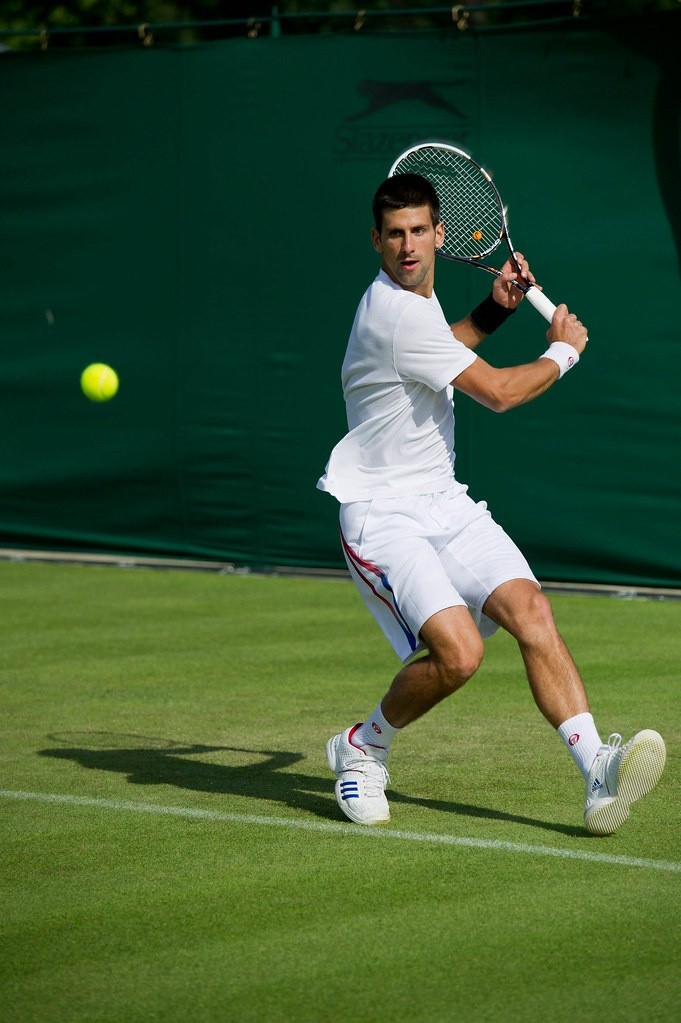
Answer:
[317,173,667,833]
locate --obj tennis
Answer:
[78,363,119,402]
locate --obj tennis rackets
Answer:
[386,141,590,343]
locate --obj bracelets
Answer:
[540,341,579,379]
[471,292,517,334]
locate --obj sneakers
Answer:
[583,729,667,833]
[324,725,394,826]
